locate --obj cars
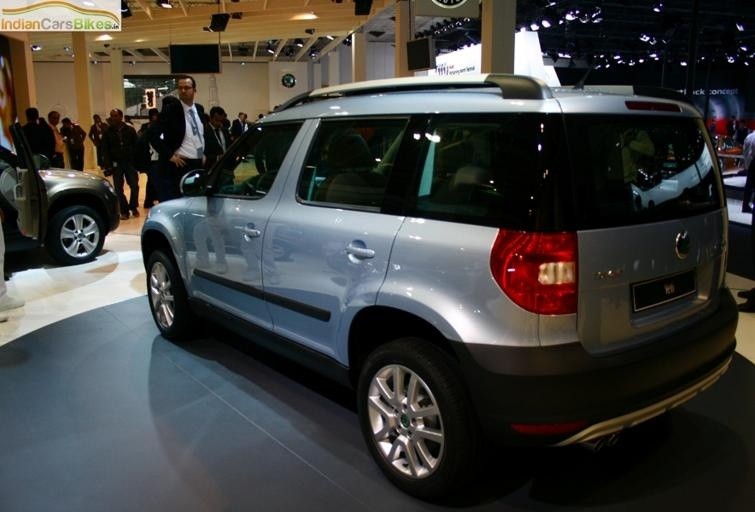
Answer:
[713,134,745,171]
[0,123,121,265]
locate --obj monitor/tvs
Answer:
[168,43,221,74]
[407,37,436,71]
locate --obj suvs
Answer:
[141,73,738,501]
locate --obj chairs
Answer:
[322,129,383,208]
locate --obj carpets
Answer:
[1,285,755,510]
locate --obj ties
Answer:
[215,128,223,144]
[188,109,203,159]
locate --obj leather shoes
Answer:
[120,207,140,220]
[736,287,755,313]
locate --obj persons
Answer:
[0,221,25,325]
[22,76,264,216]
[710,118,718,135]
[731,114,755,314]
